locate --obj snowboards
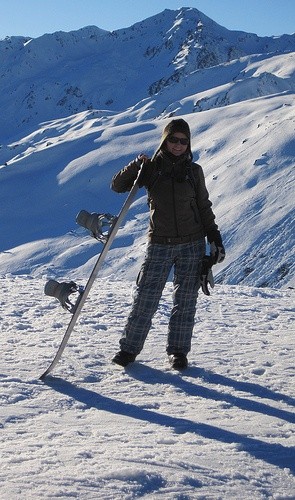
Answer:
[38,159,146,380]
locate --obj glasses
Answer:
[168,136,189,145]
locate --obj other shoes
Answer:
[169,354,189,370]
[112,350,136,367]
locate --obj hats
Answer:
[150,118,193,162]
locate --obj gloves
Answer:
[209,234,225,263]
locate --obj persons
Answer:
[109,119,225,370]
